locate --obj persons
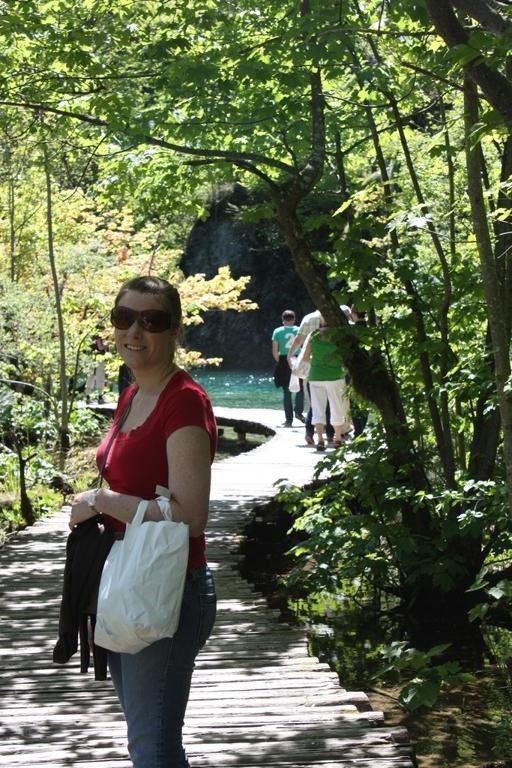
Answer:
[83,325,108,405]
[272,298,371,453]
[58,275,214,766]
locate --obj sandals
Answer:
[317,441,327,451]
[333,440,343,450]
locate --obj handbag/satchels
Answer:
[94,496,191,656]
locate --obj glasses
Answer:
[109,306,172,334]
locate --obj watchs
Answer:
[86,488,101,515]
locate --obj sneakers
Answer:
[305,433,314,445]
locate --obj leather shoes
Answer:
[295,413,305,423]
[281,421,292,427]
